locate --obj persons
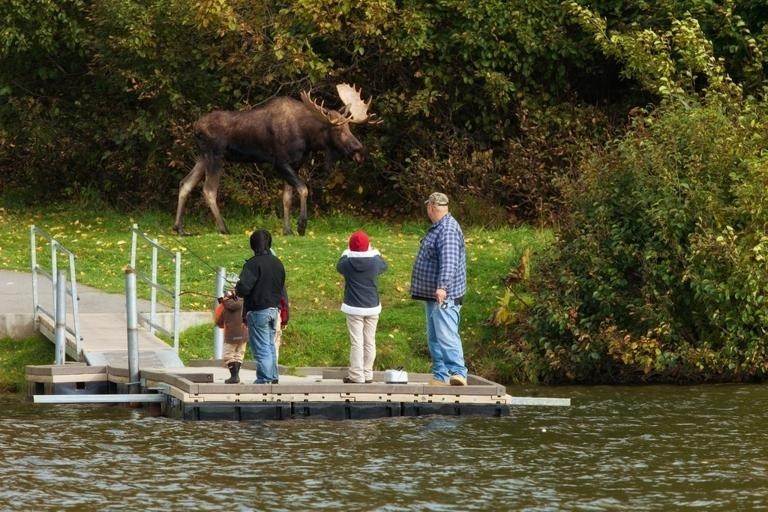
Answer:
[242,248,289,367]
[336,230,388,383]
[410,192,468,385]
[222,280,248,383]
[235,230,285,384]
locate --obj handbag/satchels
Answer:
[384,370,408,382]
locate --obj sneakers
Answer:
[343,377,373,383]
[430,374,467,385]
[254,379,278,384]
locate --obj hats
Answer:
[224,272,240,291]
[349,232,369,250]
[424,192,449,204]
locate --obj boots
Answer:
[224,362,241,384]
